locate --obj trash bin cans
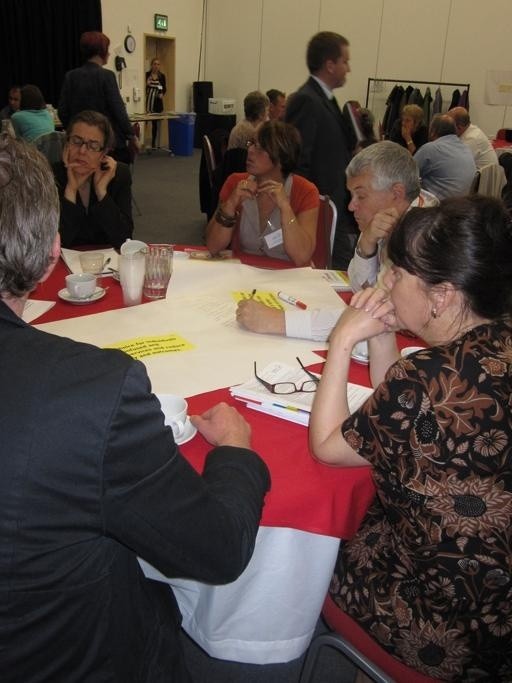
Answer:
[167,111,197,158]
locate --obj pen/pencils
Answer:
[102,258,111,270]
[250,289,256,299]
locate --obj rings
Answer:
[237,309,241,316]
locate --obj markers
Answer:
[277,291,307,310]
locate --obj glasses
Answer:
[68,135,105,151]
[245,138,267,151]
[254,358,319,394]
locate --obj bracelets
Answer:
[356,239,378,258]
[281,216,297,226]
[215,206,240,227]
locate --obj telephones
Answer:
[115,56,127,71]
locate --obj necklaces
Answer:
[259,205,276,226]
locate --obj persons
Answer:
[283,31,360,270]
[144,59,168,149]
[234,139,445,342]
[228,89,288,147]
[203,120,323,266]
[345,101,498,202]
[307,196,512,682]
[0,140,271,682]
[1,32,136,251]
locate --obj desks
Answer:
[128,112,182,157]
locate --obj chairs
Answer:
[203,136,218,188]
[309,194,337,269]
[471,163,506,195]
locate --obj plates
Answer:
[58,286,105,304]
[171,416,197,445]
[401,346,426,357]
[351,341,369,364]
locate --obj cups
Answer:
[142,246,173,298]
[80,251,104,276]
[118,254,143,304]
[160,396,188,437]
[64,274,97,297]
